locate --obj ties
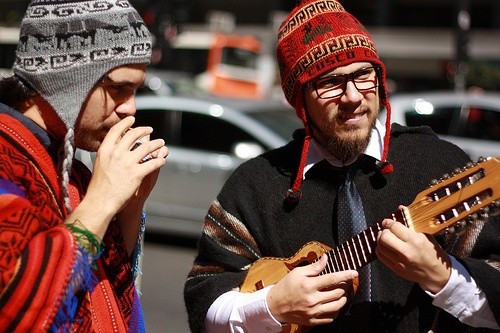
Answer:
[337,168,371,304]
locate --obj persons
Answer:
[0,0,169,333]
[184,0,500,333]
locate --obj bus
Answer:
[172,29,263,100]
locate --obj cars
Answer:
[379,91,500,164]
[133,71,212,98]
[73,93,304,237]
[1,26,21,78]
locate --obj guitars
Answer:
[239,152,495,333]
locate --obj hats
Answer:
[10,0,153,216]
[275,0,394,202]
